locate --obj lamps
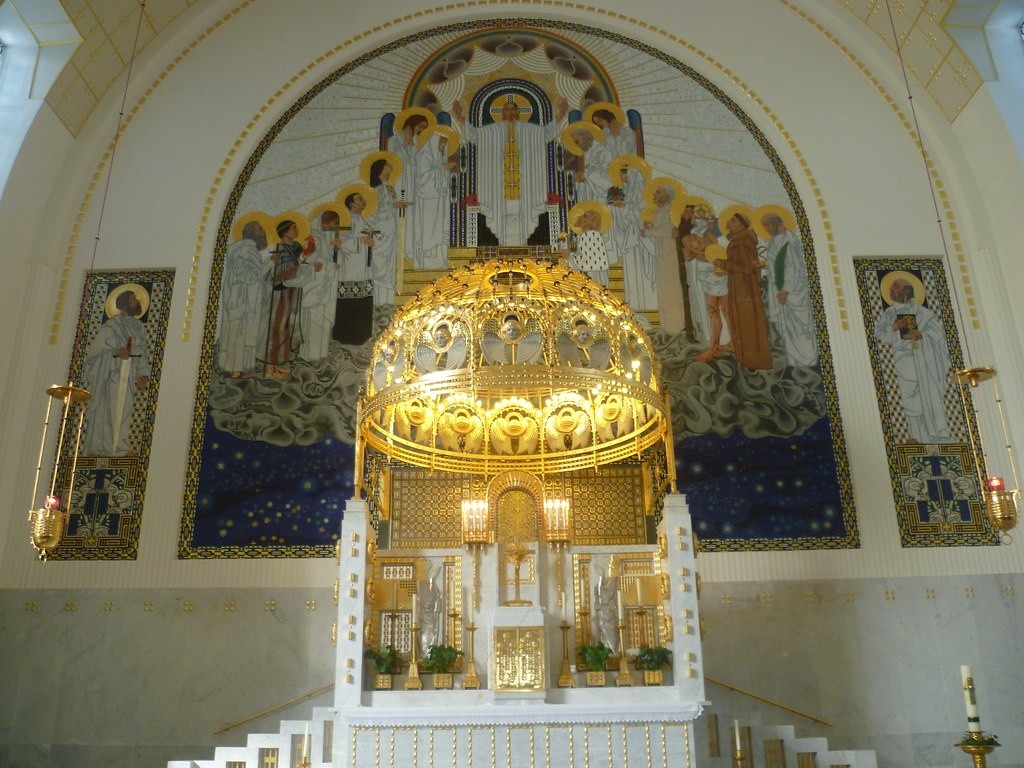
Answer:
[546,499,571,541]
[463,500,489,543]
[27,381,92,563]
[952,364,1023,546]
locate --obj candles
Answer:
[735,719,741,750]
[961,665,982,732]
[412,594,418,624]
[470,593,474,623]
[637,578,643,606]
[618,590,623,619]
[581,579,585,607]
[392,583,396,610]
[451,581,454,609]
[304,723,309,757]
[562,591,567,620]
